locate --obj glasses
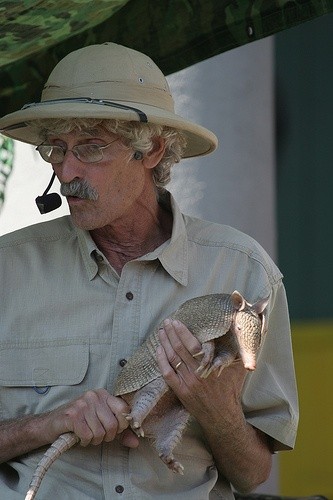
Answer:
[38,130,131,167]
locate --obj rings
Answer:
[173,360,184,371]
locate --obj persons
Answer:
[1,41,301,500]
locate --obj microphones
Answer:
[33,190,64,216]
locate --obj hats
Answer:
[2,40,216,161]
